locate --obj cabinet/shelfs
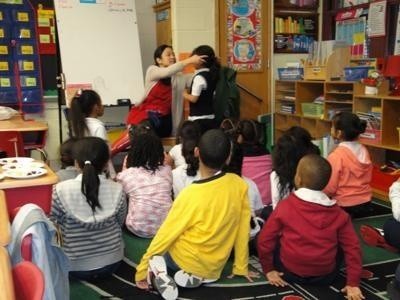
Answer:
[274,78,400,203]
[273,1,320,54]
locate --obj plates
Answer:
[0,157,48,179]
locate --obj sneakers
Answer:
[174,269,218,288]
[359,225,400,254]
[147,254,179,300]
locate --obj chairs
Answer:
[9,204,37,262]
[13,260,46,300]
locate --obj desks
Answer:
[0,115,49,157]
[1,157,61,223]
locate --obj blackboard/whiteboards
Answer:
[53,0,145,107]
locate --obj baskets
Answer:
[304,66,327,80]
[278,68,303,79]
[343,67,376,81]
[301,103,324,116]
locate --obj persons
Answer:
[109,43,210,164]
[114,119,264,300]
[359,176,400,251]
[181,45,221,137]
[47,88,127,281]
[255,108,374,300]
